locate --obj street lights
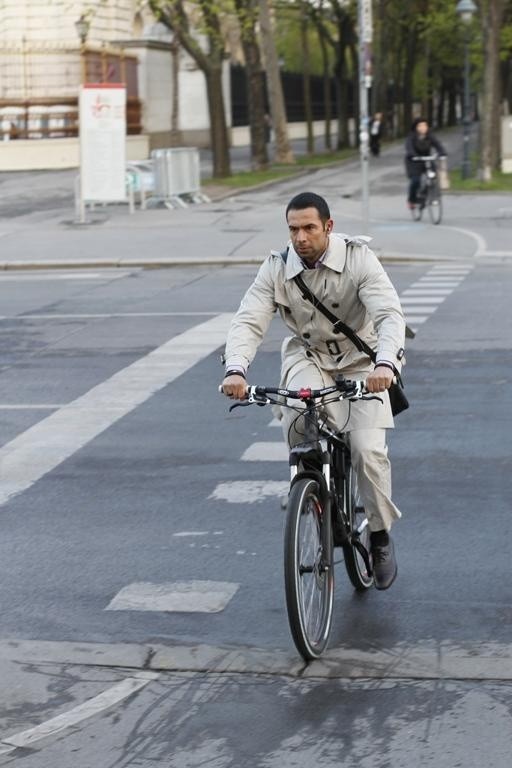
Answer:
[457,0,478,180]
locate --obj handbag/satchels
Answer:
[388,384,409,417]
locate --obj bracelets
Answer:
[223,371,245,378]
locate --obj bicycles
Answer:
[216,371,401,660]
[405,151,448,225]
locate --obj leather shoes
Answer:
[372,536,397,590]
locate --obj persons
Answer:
[221,193,407,591]
[404,118,448,209]
[368,112,384,154]
